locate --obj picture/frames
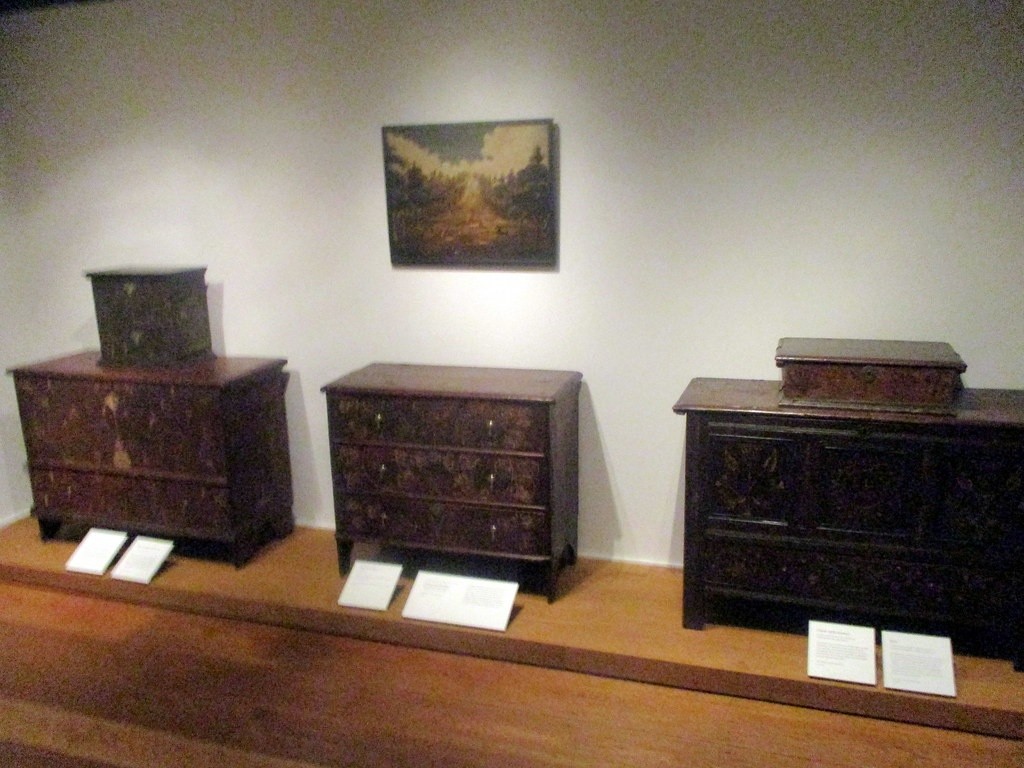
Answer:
[379,116,559,270]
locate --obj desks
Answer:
[1,506,1023,765]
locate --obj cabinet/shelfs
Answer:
[672,375,1024,674]
[7,349,299,569]
[86,267,218,369]
[320,363,582,601]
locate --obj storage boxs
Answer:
[775,334,968,415]
